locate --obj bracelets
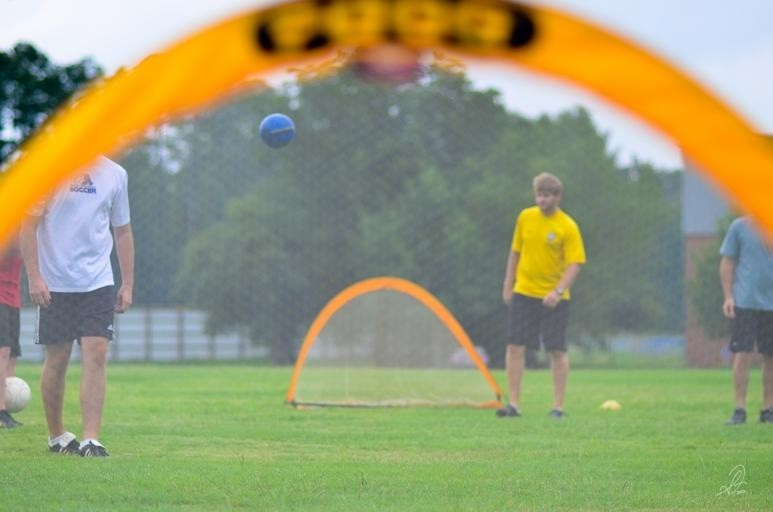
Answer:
[555,286,564,296]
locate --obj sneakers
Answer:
[46,431,110,460]
[543,409,565,419]
[722,409,749,425]
[759,409,772,424]
[496,405,522,418]
[1,409,22,425]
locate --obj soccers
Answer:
[259,112,294,148]
[5,377,30,412]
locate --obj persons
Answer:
[719,215,772,425]
[20,152,136,458]
[496,172,586,420]
[0,234,24,429]
